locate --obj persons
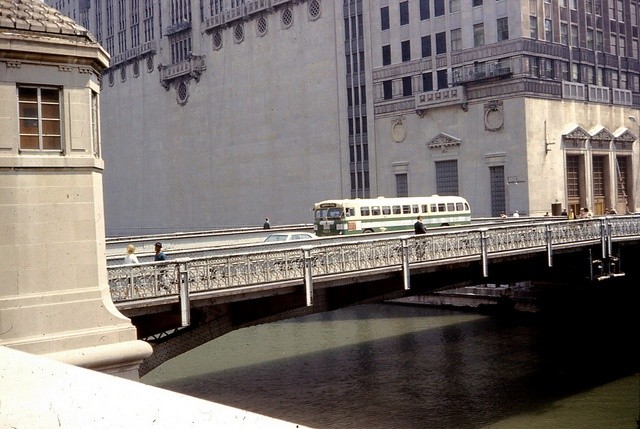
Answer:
[122,244,143,299]
[580,208,591,219]
[415,215,428,260]
[153,241,172,294]
[500,210,506,218]
[263,217,269,229]
[513,210,519,218]
[561,208,567,216]
[544,212,549,216]
[568,209,574,220]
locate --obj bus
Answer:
[308,193,472,238]
